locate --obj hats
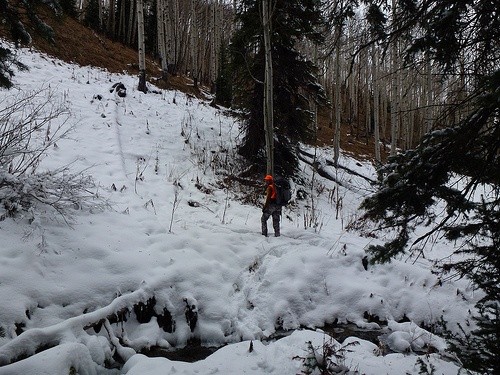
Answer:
[264,175,273,181]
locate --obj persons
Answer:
[261,175,281,238]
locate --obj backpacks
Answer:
[277,179,292,204]
